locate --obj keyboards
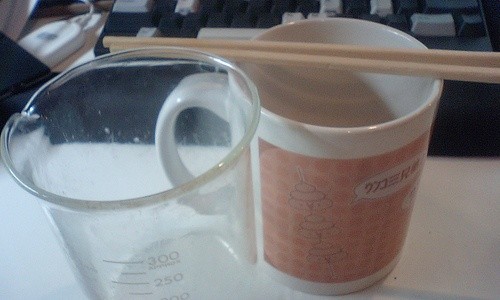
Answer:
[94,0,494,60]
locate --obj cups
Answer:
[0,44,260,300]
[154,17,444,295]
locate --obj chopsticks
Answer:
[102,33,500,97]
